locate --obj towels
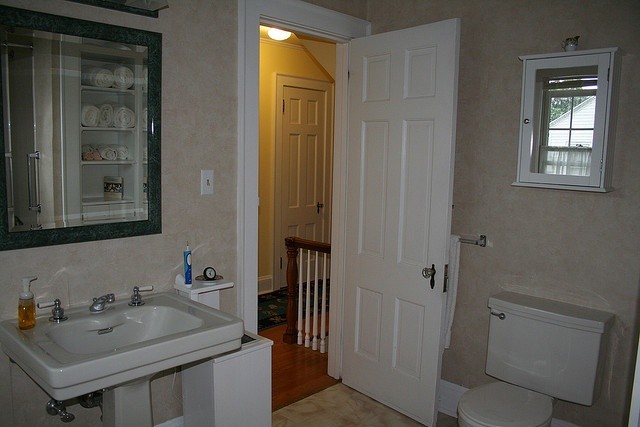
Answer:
[82,65,115,88]
[83,144,93,161]
[98,104,114,127]
[96,145,117,161]
[444,236,461,350]
[100,64,135,89]
[111,103,135,128]
[81,105,100,128]
[107,144,128,160]
[91,145,103,161]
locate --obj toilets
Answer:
[456,292,615,427]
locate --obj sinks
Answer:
[43,304,206,356]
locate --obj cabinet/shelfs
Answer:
[0,5,162,253]
[180,336,274,427]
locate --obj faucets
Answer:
[88,293,116,312]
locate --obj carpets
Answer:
[258,272,332,332]
